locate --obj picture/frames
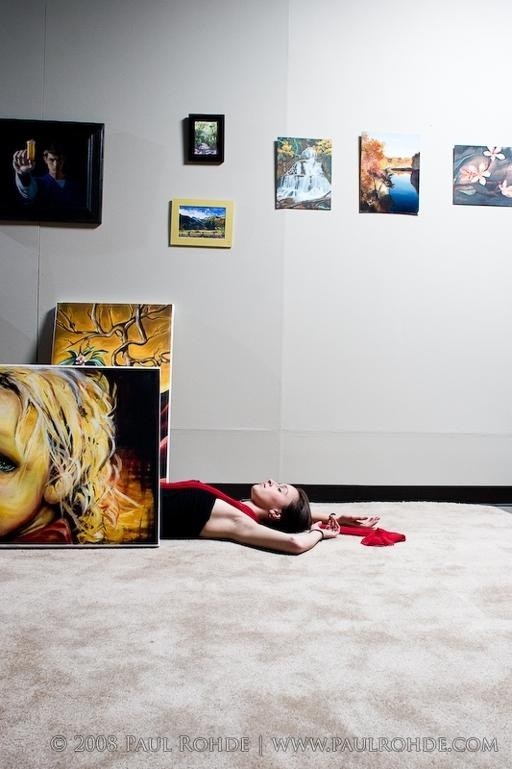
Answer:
[169,198,235,248]
[0,118,105,227]
[188,114,225,165]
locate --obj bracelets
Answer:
[308,529,323,541]
[328,511,335,521]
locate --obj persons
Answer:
[1,365,147,546]
[160,478,382,556]
[13,144,82,220]
